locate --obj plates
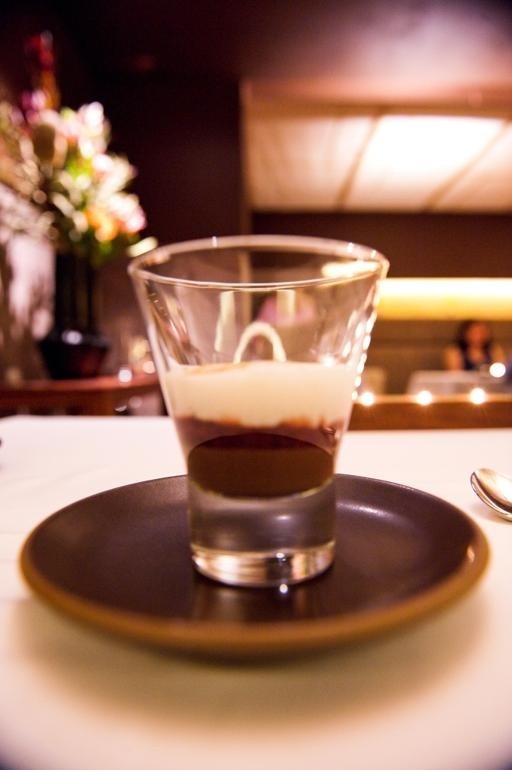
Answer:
[20,474,490,666]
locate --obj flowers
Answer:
[0,87,158,258]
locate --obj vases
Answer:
[36,249,114,379]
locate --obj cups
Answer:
[127,232,388,590]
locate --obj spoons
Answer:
[470,467,512,521]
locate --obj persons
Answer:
[445,320,507,374]
